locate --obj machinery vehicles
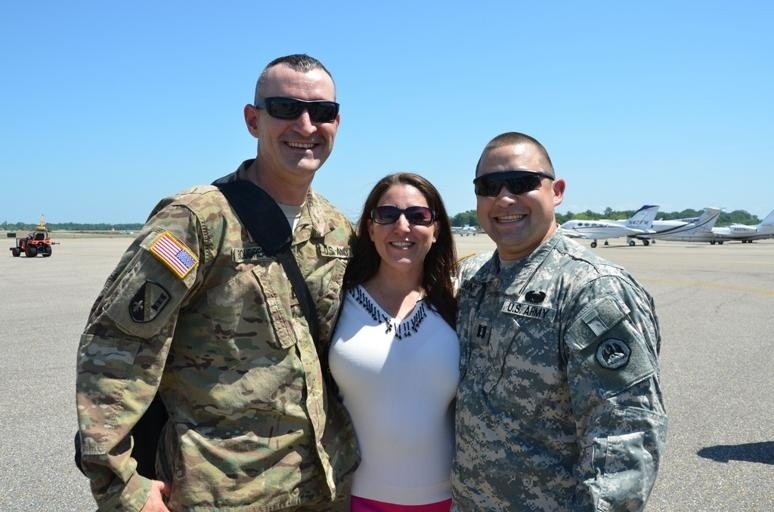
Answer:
[10,214,52,257]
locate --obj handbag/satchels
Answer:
[75,392,170,479]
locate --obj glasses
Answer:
[473,171,554,197]
[258,94,340,124]
[369,205,436,226]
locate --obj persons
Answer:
[449,131,668,511]
[323,172,466,511]
[70,53,459,511]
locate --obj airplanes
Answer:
[561,206,774,248]
